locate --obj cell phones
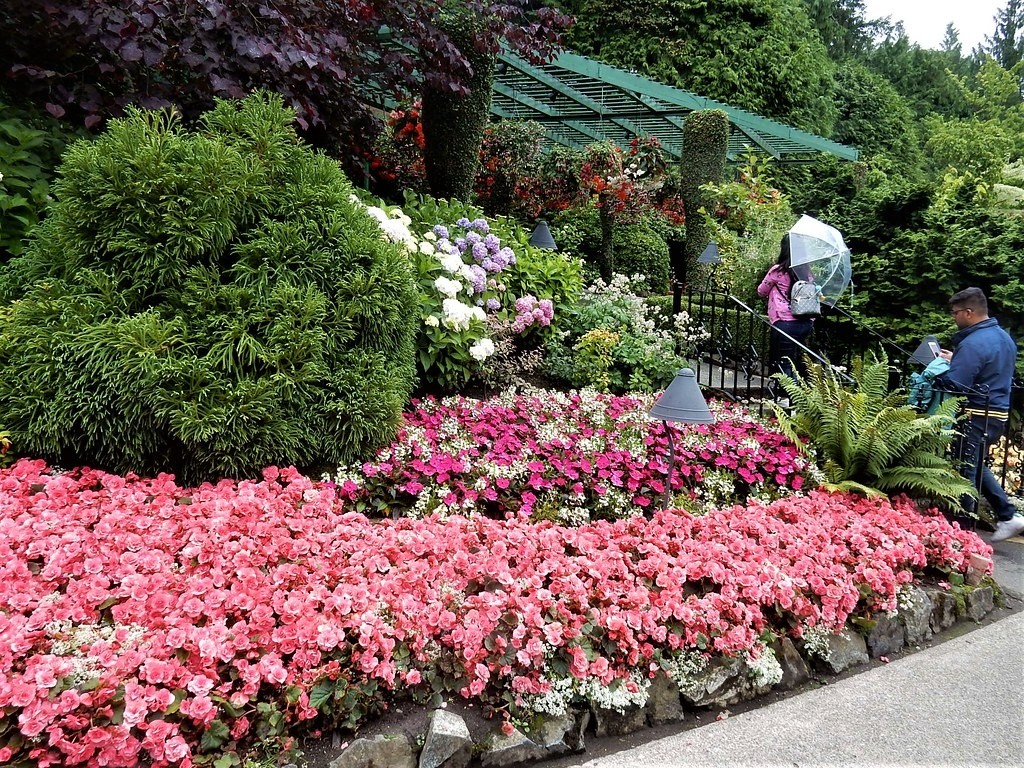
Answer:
[928,342,940,359]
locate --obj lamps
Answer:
[529,221,558,254]
[648,367,716,509]
[696,242,722,290]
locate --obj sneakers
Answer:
[991,512,1024,542]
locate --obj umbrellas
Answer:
[787,214,852,309]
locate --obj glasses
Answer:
[950,307,973,315]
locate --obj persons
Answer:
[934,287,1024,542]
[757,234,815,416]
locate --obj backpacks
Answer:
[789,278,822,317]
